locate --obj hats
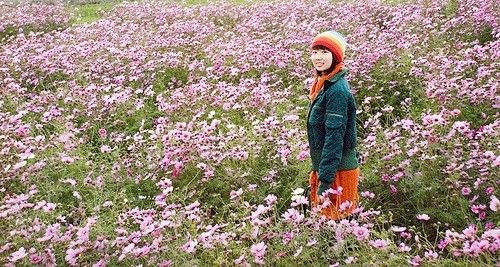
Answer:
[311,31,347,64]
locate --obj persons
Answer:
[306,29,360,247]
